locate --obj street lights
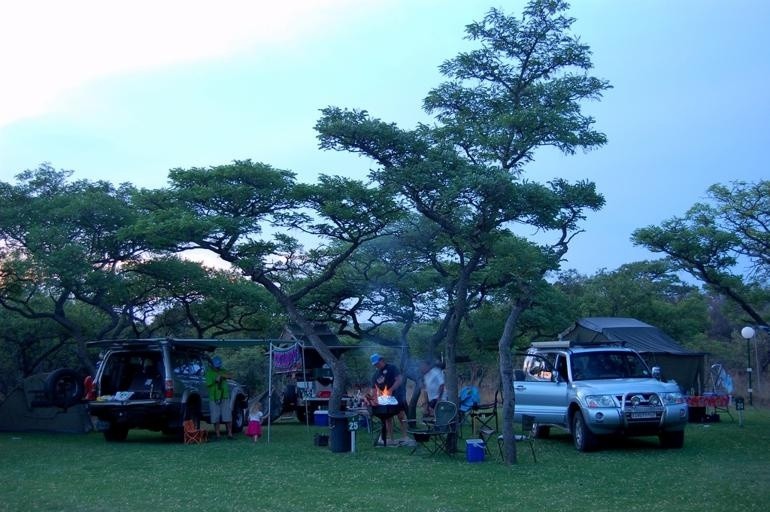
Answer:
[740,322,758,408]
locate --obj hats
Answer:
[370,353,384,365]
[212,357,221,368]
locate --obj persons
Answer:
[416,358,447,417]
[368,352,409,445]
[205,355,236,441]
[245,401,265,442]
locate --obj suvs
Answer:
[511,338,688,449]
[42,343,248,441]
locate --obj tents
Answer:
[1,368,113,434]
[559,317,706,395]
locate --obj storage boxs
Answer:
[313,409,329,426]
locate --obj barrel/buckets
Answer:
[328,410,358,453]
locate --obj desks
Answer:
[684,393,734,421]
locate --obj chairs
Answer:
[405,384,539,464]
[183,419,208,444]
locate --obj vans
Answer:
[290,359,347,423]
